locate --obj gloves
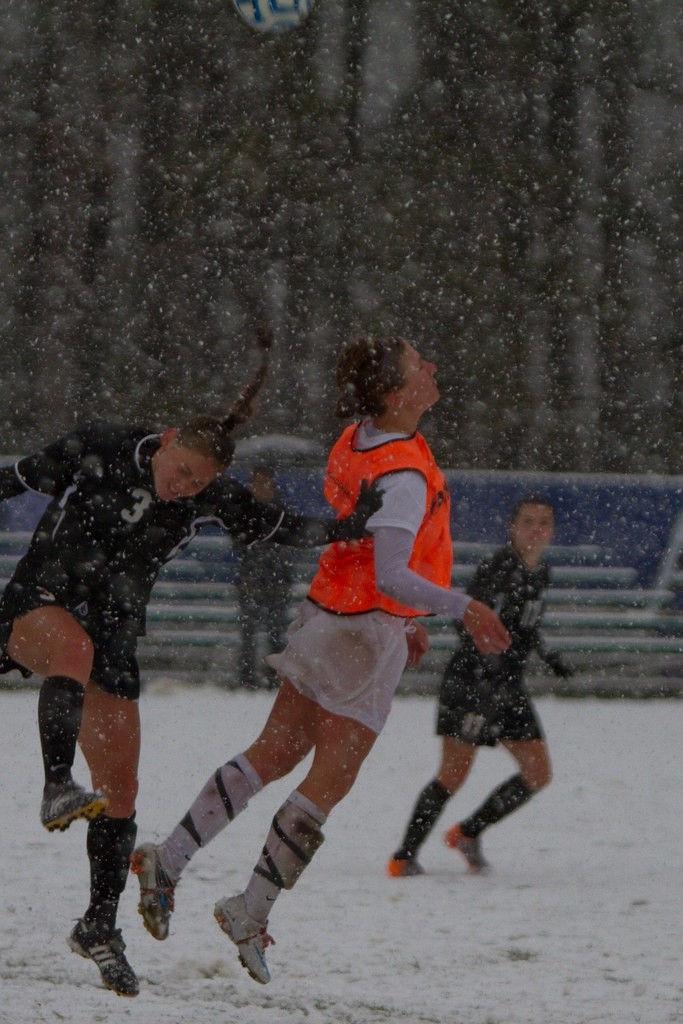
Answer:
[544,648,576,679]
[336,479,385,541]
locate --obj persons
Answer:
[389,496,573,882]
[219,472,294,691]
[0,328,384,995]
[129,336,512,985]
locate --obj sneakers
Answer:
[445,822,490,876]
[40,781,110,832]
[67,918,139,997]
[215,893,275,984]
[131,842,182,941]
[388,855,424,877]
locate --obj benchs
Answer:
[0,530,683,694]
